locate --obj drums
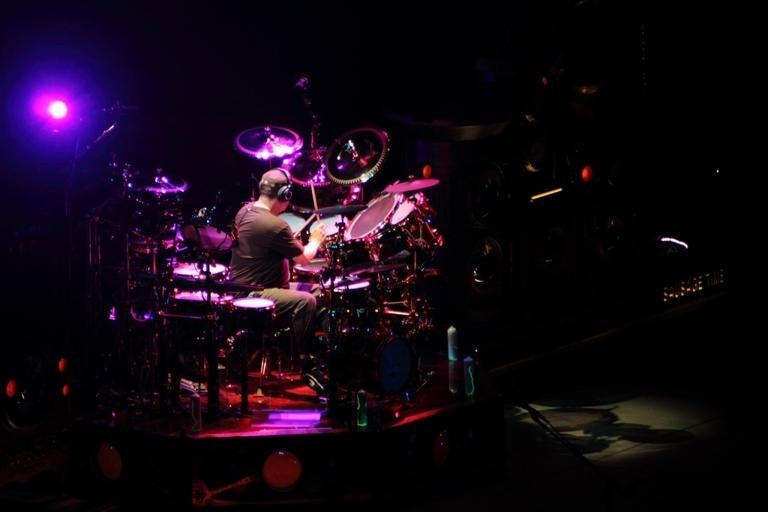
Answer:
[167,292,225,344]
[345,194,443,270]
[178,223,231,264]
[126,243,164,284]
[232,298,277,331]
[277,212,309,244]
[296,263,344,283]
[335,283,374,312]
[335,323,418,398]
[310,215,350,258]
[391,195,437,237]
[164,259,231,292]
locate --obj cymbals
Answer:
[383,178,441,194]
[128,167,192,194]
[327,128,388,183]
[281,146,331,186]
[237,126,304,162]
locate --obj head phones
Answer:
[273,167,293,202]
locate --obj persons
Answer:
[232,167,316,355]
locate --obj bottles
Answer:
[463,356,476,397]
[190,392,203,433]
[353,389,370,429]
[447,325,459,362]
